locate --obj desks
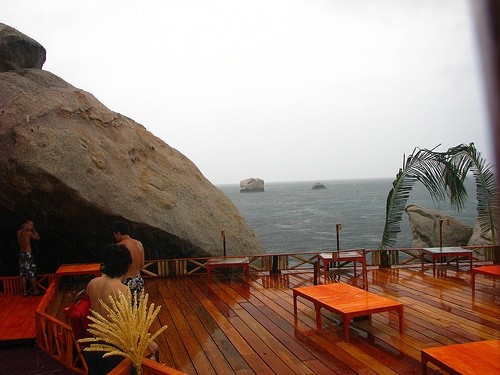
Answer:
[422,247,473,277]
[292,282,404,342]
[471,265,500,290]
[421,338,500,375]
[318,252,365,283]
[208,258,250,282]
[55,264,101,283]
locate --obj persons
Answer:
[16,219,41,296]
[84,242,134,375]
[113,221,145,311]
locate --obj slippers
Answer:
[24,290,29,297]
[34,290,43,296]
